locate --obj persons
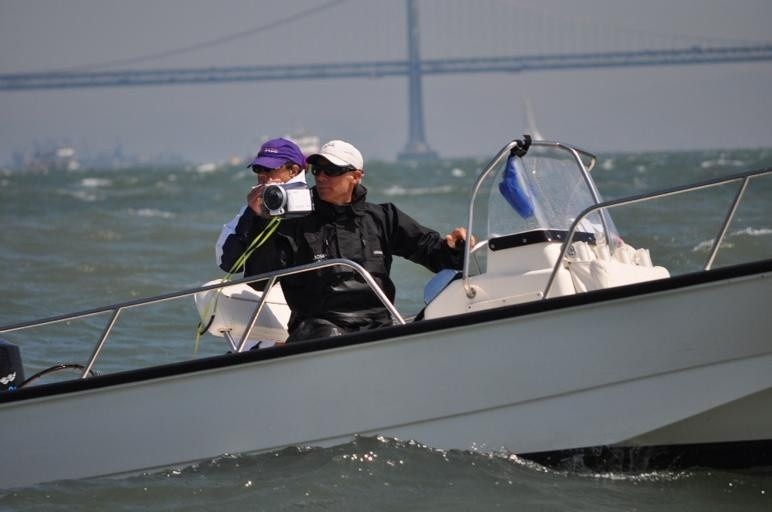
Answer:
[215,138,308,292]
[218,140,475,346]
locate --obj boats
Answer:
[0,137,772,490]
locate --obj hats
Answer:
[306,140,363,171]
[247,137,305,171]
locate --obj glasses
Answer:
[310,163,356,177]
